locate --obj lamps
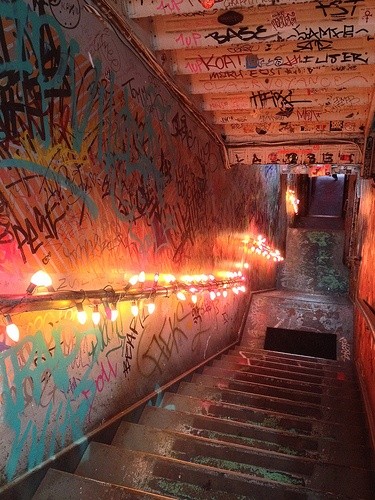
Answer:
[0,174,315,347]
